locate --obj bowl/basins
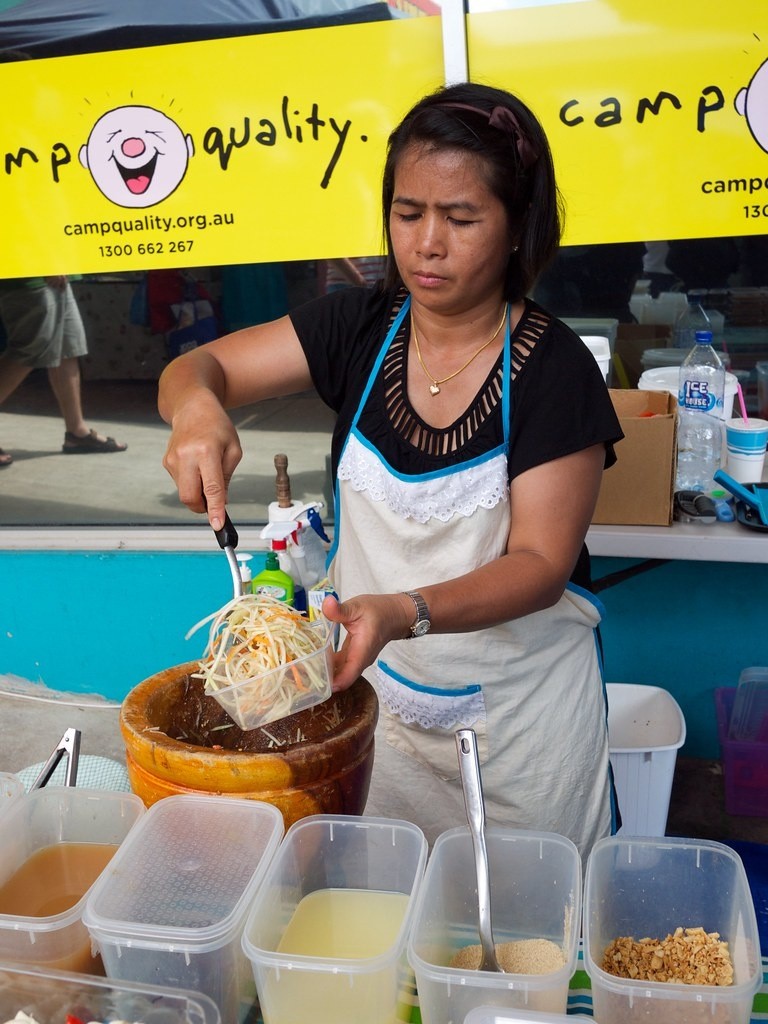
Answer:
[120,657,380,826]
[1,771,762,1024]
[202,616,333,729]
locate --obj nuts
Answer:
[597,927,737,1024]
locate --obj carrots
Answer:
[203,606,311,715]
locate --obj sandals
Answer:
[0,448,13,465]
[61,428,128,453]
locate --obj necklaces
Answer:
[412,303,508,396]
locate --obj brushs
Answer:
[706,490,735,522]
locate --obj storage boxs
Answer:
[715,683,768,815]
[589,389,679,527]
[1,771,762,1023]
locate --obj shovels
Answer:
[451,728,527,1016]
[202,486,259,657]
[714,469,768,525]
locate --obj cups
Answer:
[725,417,768,484]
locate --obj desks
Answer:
[582,520,768,611]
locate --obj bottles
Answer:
[271,538,301,587]
[673,290,712,348]
[673,330,726,493]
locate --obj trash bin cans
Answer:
[605,682,686,871]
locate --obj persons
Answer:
[0,274,128,465]
[157,81,622,853]
[325,256,386,291]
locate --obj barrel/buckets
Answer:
[640,348,731,371]
[637,366,739,421]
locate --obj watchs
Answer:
[402,591,431,640]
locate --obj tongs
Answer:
[23,727,82,810]
